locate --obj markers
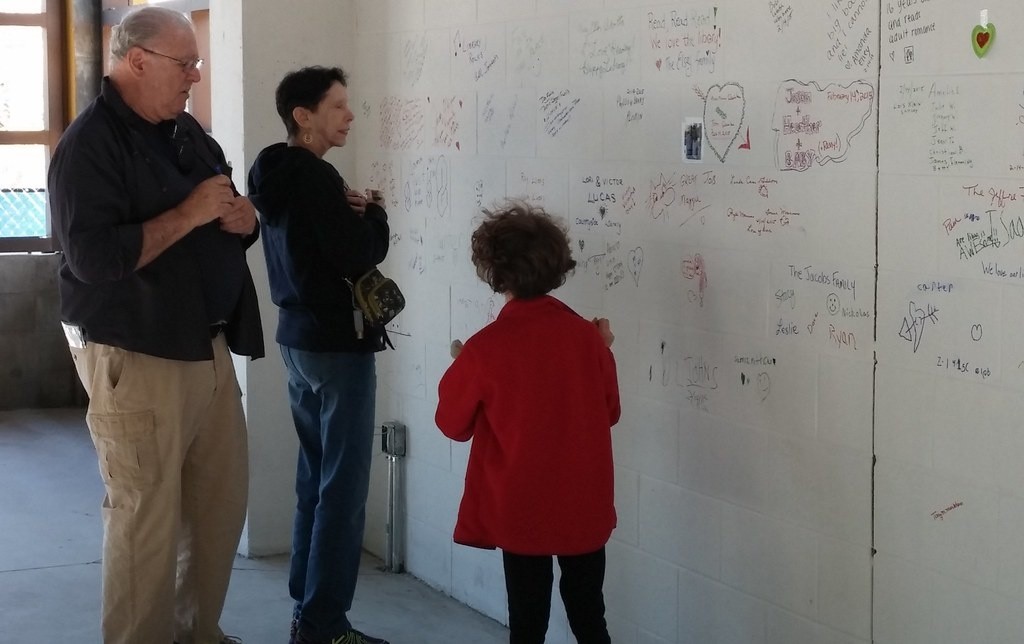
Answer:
[596,320,599,328]
[372,197,381,200]
[215,164,223,175]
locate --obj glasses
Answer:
[135,45,204,72]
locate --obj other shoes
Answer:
[289,619,389,644]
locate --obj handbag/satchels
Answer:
[355,266,407,331]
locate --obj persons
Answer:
[249,65,392,644]
[48,8,265,644]
[435,197,621,644]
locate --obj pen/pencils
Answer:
[228,161,232,168]
